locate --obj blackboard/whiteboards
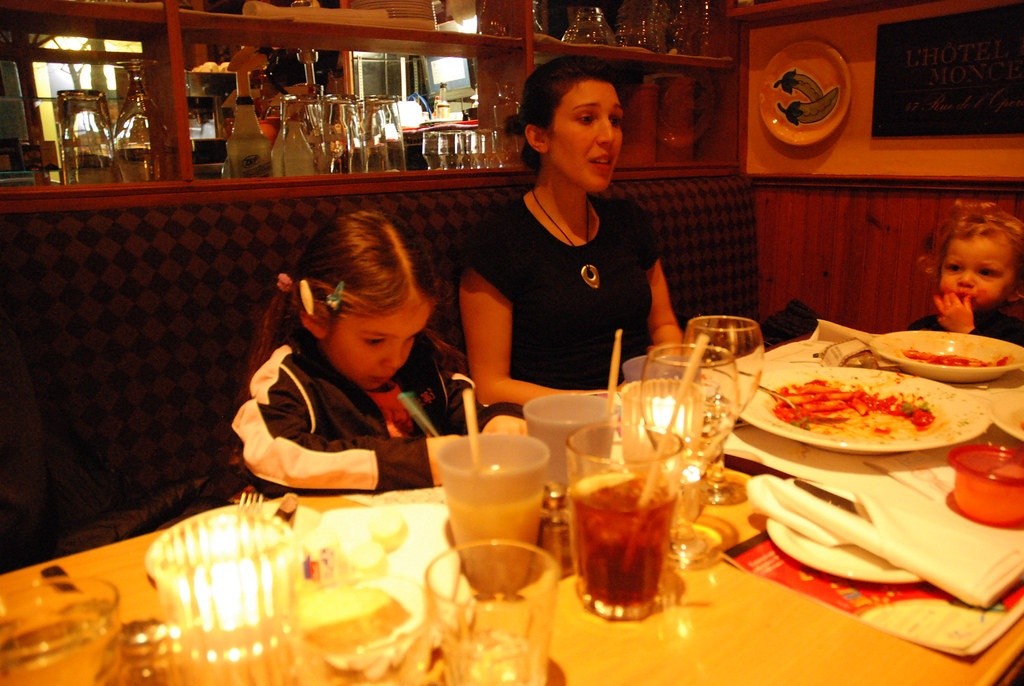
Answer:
[870,1,1024,138]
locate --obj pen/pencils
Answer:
[395,392,439,441]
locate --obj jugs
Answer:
[147,512,300,686]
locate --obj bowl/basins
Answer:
[948,447,1024,525]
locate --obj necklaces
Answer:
[531,189,600,288]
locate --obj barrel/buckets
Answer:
[617,83,657,164]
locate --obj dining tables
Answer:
[0,333,1024,686]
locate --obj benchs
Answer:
[0,176,756,579]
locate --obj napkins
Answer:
[747,426,1024,612]
[788,318,903,370]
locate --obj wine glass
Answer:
[646,347,743,568]
[685,316,764,505]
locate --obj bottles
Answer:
[437,82,451,120]
[492,83,521,166]
[117,619,164,685]
[541,487,577,581]
[114,60,172,182]
[560,0,713,59]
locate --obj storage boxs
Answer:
[191,139,228,165]
[0,137,26,179]
[185,69,238,100]
[22,145,43,171]
[40,141,59,170]
[403,125,460,171]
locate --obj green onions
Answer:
[901,402,930,416]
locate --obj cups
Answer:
[566,424,684,620]
[422,132,496,169]
[56,90,120,184]
[439,433,548,592]
[0,577,121,686]
[622,354,698,383]
[523,398,617,487]
[281,94,406,175]
[426,540,558,686]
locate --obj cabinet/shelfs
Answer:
[0,0,741,201]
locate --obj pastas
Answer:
[783,384,870,417]
[904,350,982,368]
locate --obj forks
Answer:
[239,494,264,508]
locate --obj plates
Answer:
[870,330,1024,382]
[989,388,1024,441]
[766,516,927,583]
[354,0,434,21]
[759,41,851,146]
[724,364,992,453]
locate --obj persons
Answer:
[909,198,1024,349]
[460,57,684,404]
[232,210,528,493]
